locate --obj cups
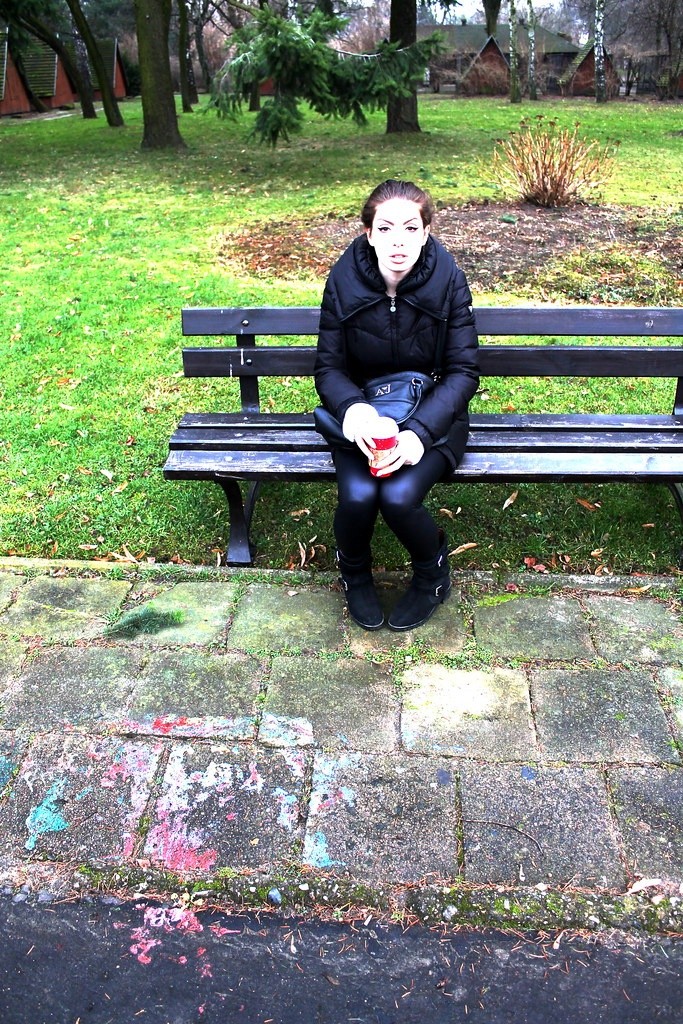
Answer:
[365,416,398,477]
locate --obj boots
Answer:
[386,527,453,631]
[334,545,385,629]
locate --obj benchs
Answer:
[161,306,683,564]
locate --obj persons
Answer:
[313,179,481,631]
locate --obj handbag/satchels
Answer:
[312,369,451,452]
